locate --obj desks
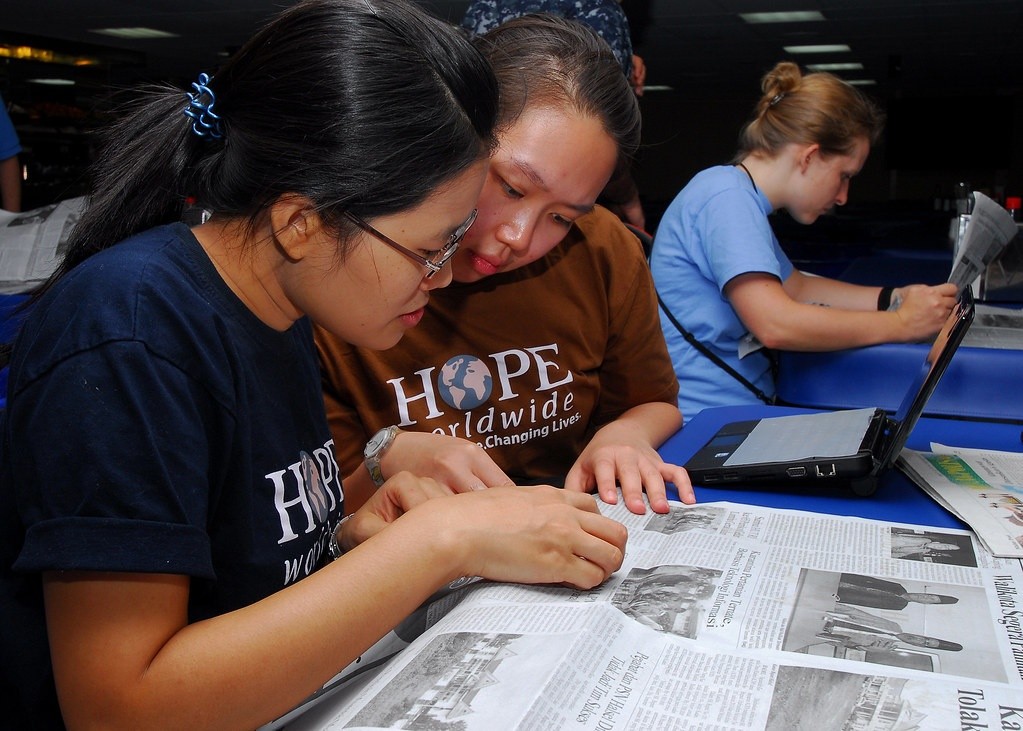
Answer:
[776,342,1023,424]
[318,406,1023,731]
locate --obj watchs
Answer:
[363,426,406,487]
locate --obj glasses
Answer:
[335,207,479,278]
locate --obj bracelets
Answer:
[329,512,355,559]
[877,286,894,311]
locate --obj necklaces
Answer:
[738,161,757,194]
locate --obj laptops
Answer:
[681,282,973,486]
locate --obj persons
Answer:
[978,492,1023,524]
[826,603,963,652]
[456,0,646,239]
[0,0,627,731]
[315,14,697,515]
[835,573,959,611]
[891,531,960,558]
[0,96,43,407]
[623,573,699,629]
[648,59,959,424]
